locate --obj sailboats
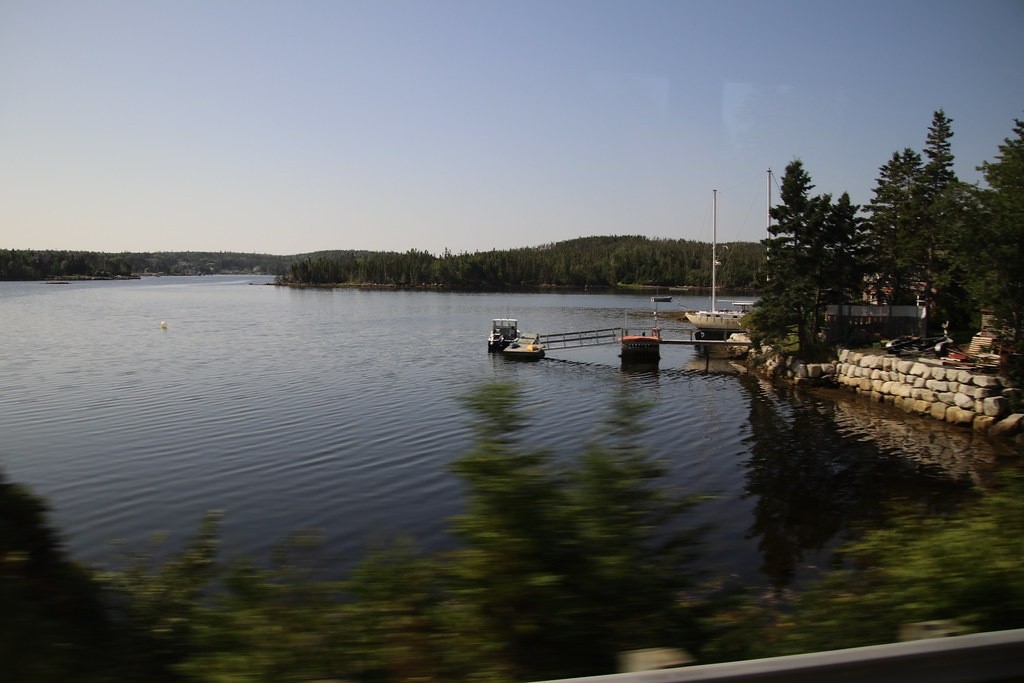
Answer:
[684,168,787,338]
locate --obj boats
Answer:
[650,296,673,302]
[487,319,522,352]
